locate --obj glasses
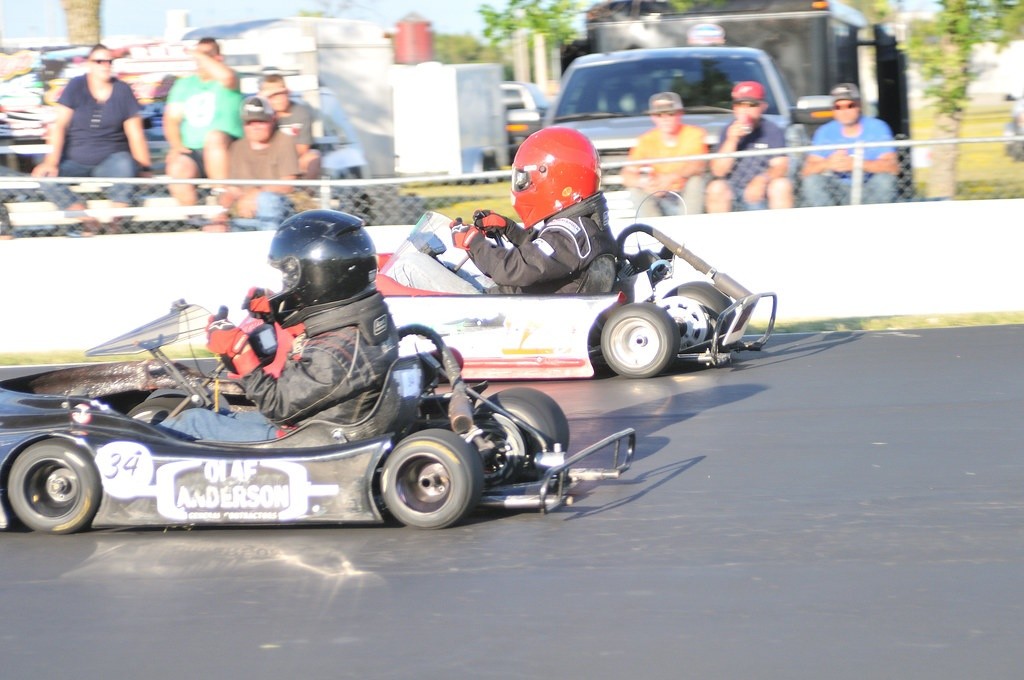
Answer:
[834,103,858,109]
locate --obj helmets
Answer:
[269,209,377,330]
[510,127,601,229]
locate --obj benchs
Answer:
[0,138,366,231]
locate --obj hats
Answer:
[731,81,765,103]
[831,83,859,100]
[265,82,287,98]
[645,92,686,114]
[257,67,282,83]
[243,99,272,122]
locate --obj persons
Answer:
[164,38,321,232]
[387,127,617,295]
[705,81,794,212]
[31,45,152,236]
[160,210,398,443]
[619,94,709,217]
[799,84,900,205]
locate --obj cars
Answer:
[543,46,840,192]
[500,82,551,165]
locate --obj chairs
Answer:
[271,355,419,449]
[577,255,617,294]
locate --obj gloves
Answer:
[206,315,249,359]
[449,218,485,250]
[473,209,509,237]
[241,287,278,325]
